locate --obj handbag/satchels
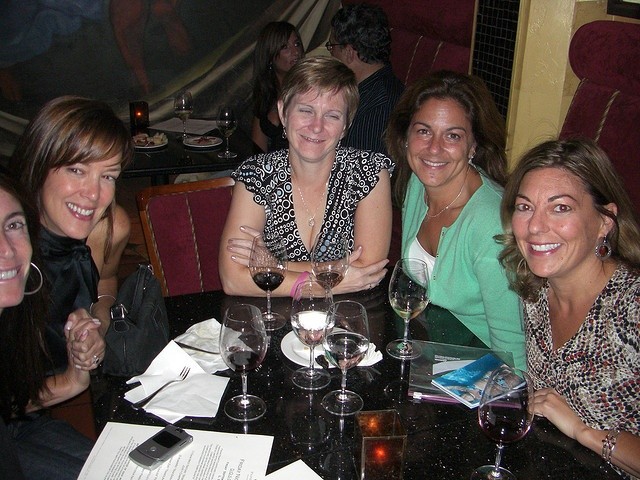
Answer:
[100,263,170,378]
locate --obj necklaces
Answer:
[420,164,469,219]
[290,155,338,229]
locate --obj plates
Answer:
[279,326,382,371]
[133,138,169,149]
[182,136,224,148]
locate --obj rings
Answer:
[369,284,372,292]
[90,354,101,364]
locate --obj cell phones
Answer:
[128,423,193,471]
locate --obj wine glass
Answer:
[174,91,193,141]
[384,257,431,361]
[289,279,334,392]
[215,104,239,158]
[470,366,536,480]
[218,303,271,423]
[321,299,371,417]
[249,232,288,333]
[310,231,351,304]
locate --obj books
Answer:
[409,340,526,409]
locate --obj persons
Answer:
[316,5,403,150]
[0,90,135,354]
[244,17,315,153]
[494,132,640,476]
[0,188,100,480]
[218,56,395,299]
[393,69,527,378]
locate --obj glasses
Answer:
[324,41,343,50]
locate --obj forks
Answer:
[130,365,191,411]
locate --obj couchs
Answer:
[378,0,476,89]
[554,19,640,233]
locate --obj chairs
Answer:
[138,176,236,296]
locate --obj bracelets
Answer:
[89,294,116,316]
[601,425,622,464]
[90,292,122,313]
